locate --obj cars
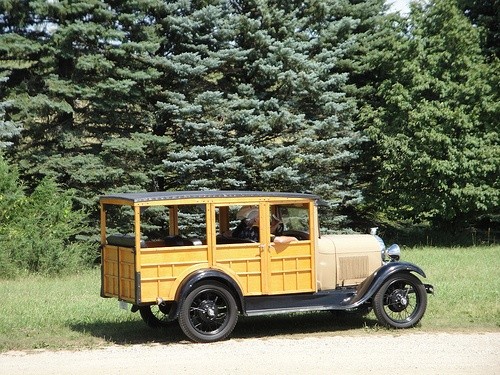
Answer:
[96,190,434,343]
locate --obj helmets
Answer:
[236,205,259,222]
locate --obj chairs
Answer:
[102,233,260,253]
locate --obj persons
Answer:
[231,205,261,239]
[247,215,300,245]
